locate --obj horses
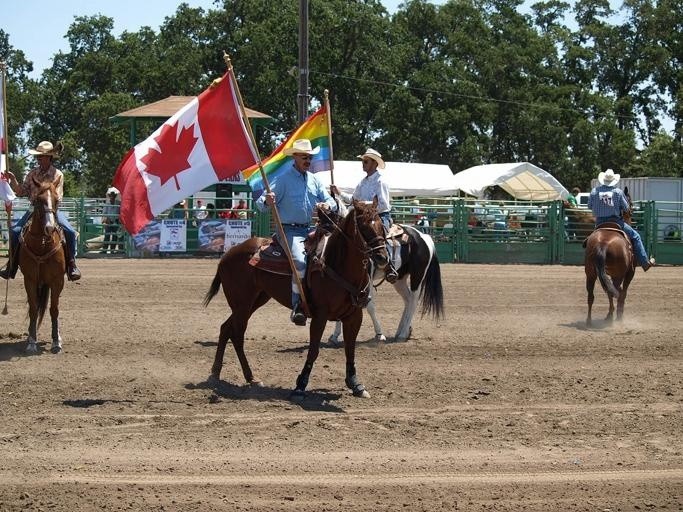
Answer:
[584,185,637,328]
[202,193,389,402]
[18,174,67,356]
[557,200,596,240]
[325,188,447,346]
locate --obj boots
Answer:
[66,258,81,280]
[1,266,16,279]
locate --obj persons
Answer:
[205,203,214,219]
[438,216,454,242]
[329,147,408,285]
[586,168,656,271]
[623,185,631,202]
[190,200,208,219]
[98,190,121,254]
[169,199,188,220]
[565,187,584,240]
[466,208,478,230]
[491,200,509,244]
[508,210,521,230]
[232,199,248,220]
[0,139,81,284]
[478,201,488,242]
[415,214,429,234]
[254,138,338,327]
[216,199,233,219]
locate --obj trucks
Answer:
[575,176,682,240]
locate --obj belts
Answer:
[293,224,308,227]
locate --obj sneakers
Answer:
[387,270,396,284]
[293,309,306,326]
[643,257,655,271]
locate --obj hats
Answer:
[283,138,321,155]
[598,168,621,187]
[356,148,385,169]
[27,141,64,155]
[107,187,120,194]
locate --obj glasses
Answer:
[301,156,312,160]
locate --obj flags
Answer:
[0,76,19,213]
[111,70,260,238]
[239,98,333,203]
